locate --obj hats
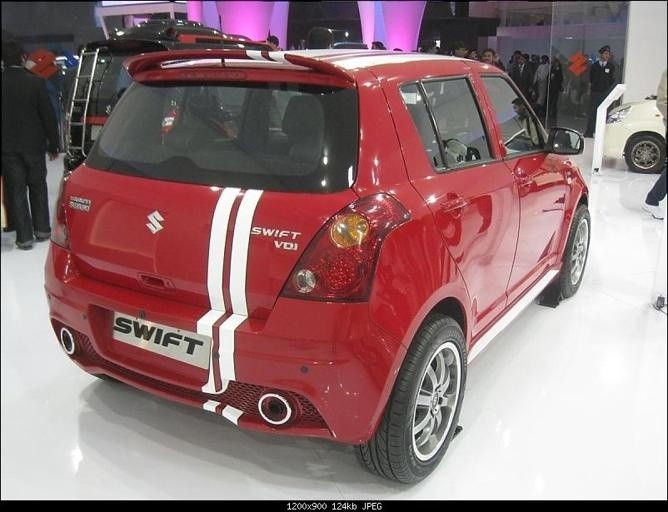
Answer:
[598,45,610,54]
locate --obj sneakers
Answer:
[641,202,665,221]
[3,221,52,250]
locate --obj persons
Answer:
[581,45,616,138]
[0,38,60,251]
[266,35,284,51]
[370,34,565,122]
[305,27,334,50]
[640,67,668,220]
[510,97,545,149]
[0,40,13,234]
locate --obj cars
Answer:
[603,99,668,174]
